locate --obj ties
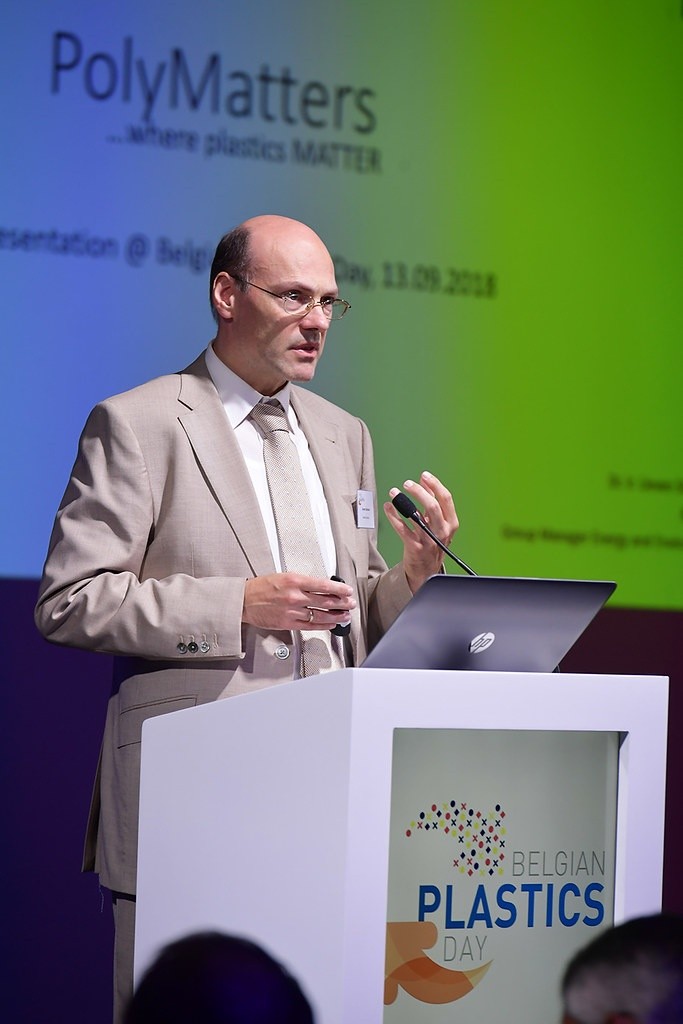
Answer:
[248,399,346,679]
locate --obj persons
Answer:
[559,909,683,1024]
[33,214,461,1024]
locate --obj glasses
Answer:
[230,275,352,320]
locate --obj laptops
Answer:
[359,575,615,672]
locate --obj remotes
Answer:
[327,576,351,636]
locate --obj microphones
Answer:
[391,493,477,576]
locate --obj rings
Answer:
[307,608,315,624]
